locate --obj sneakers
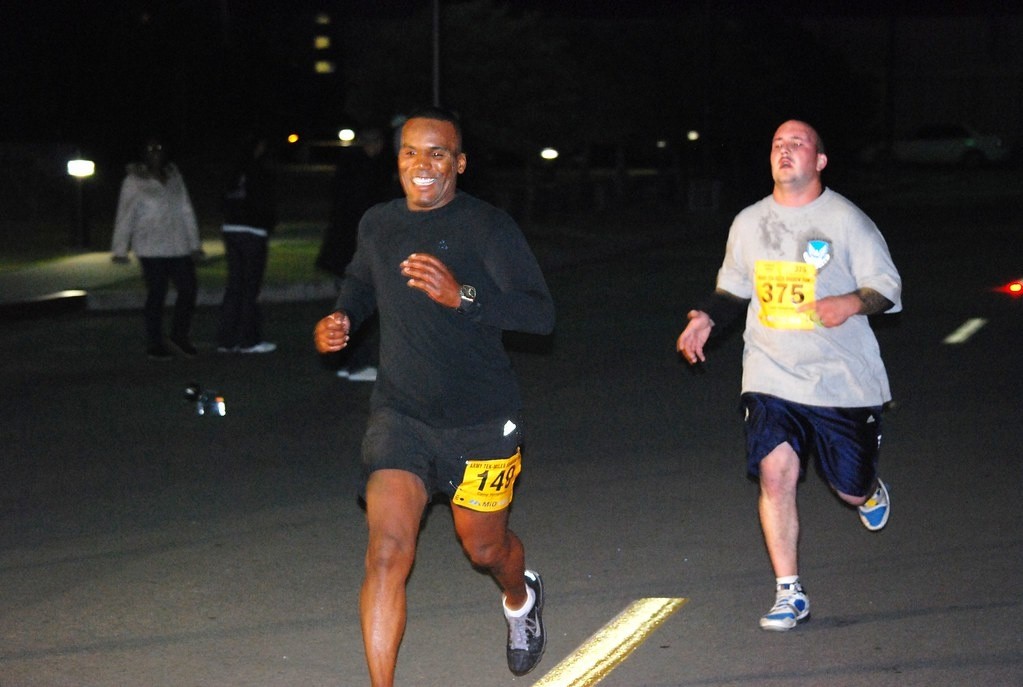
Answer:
[759,577,810,631]
[858,477,889,530]
[501,569,546,676]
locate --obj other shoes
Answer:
[349,367,377,381]
[147,347,173,361]
[169,335,196,357]
[239,340,276,353]
[336,370,350,377]
[219,345,239,353]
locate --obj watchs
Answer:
[456,284,476,313]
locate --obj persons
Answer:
[316,146,378,381]
[676,116,903,631]
[313,110,556,687]
[214,139,276,353]
[66,148,94,247]
[110,138,206,357]
